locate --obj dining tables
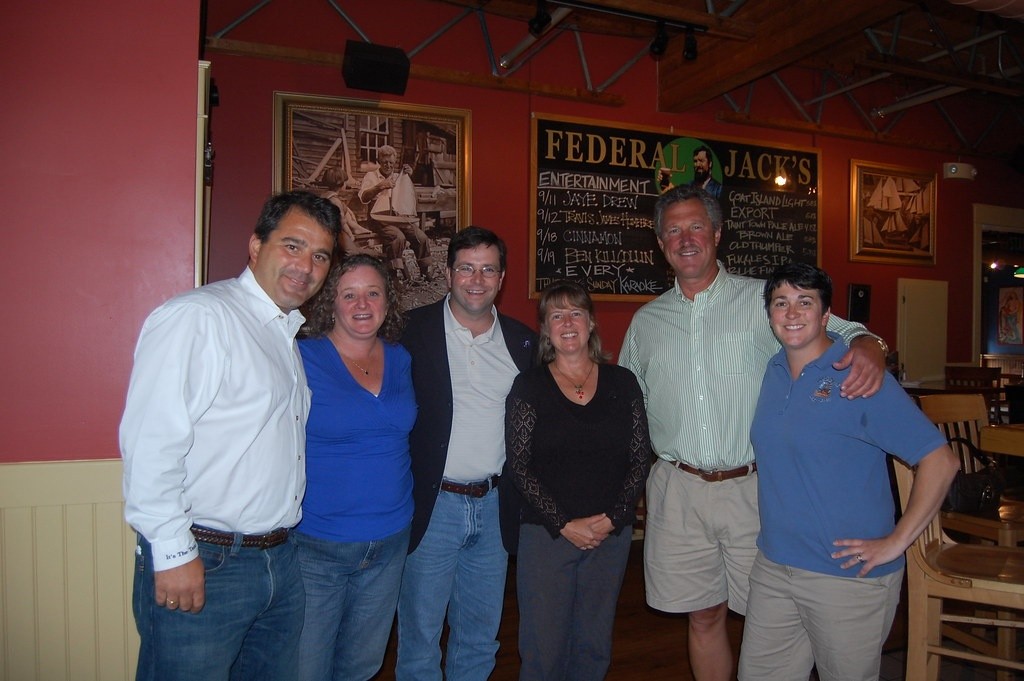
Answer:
[903,382,997,395]
[980,424,1024,457]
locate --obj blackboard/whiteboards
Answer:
[529,112,824,302]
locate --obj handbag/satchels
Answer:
[948,437,1002,521]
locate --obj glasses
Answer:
[451,263,503,279]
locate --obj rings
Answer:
[856,554,864,562]
[167,599,179,605]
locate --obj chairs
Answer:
[920,394,1024,547]
[946,366,1001,423]
[893,458,1024,681]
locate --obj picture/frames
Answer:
[849,158,938,266]
[272,91,473,335]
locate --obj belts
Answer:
[669,460,758,482]
[189,526,290,551]
[440,475,500,499]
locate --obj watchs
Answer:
[851,336,888,358]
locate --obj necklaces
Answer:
[329,332,376,375]
[553,361,594,399]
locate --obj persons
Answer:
[358,145,434,285]
[618,185,886,681]
[118,190,341,680]
[737,262,960,680]
[295,253,418,681]
[320,167,382,259]
[401,226,540,681]
[505,281,650,681]
